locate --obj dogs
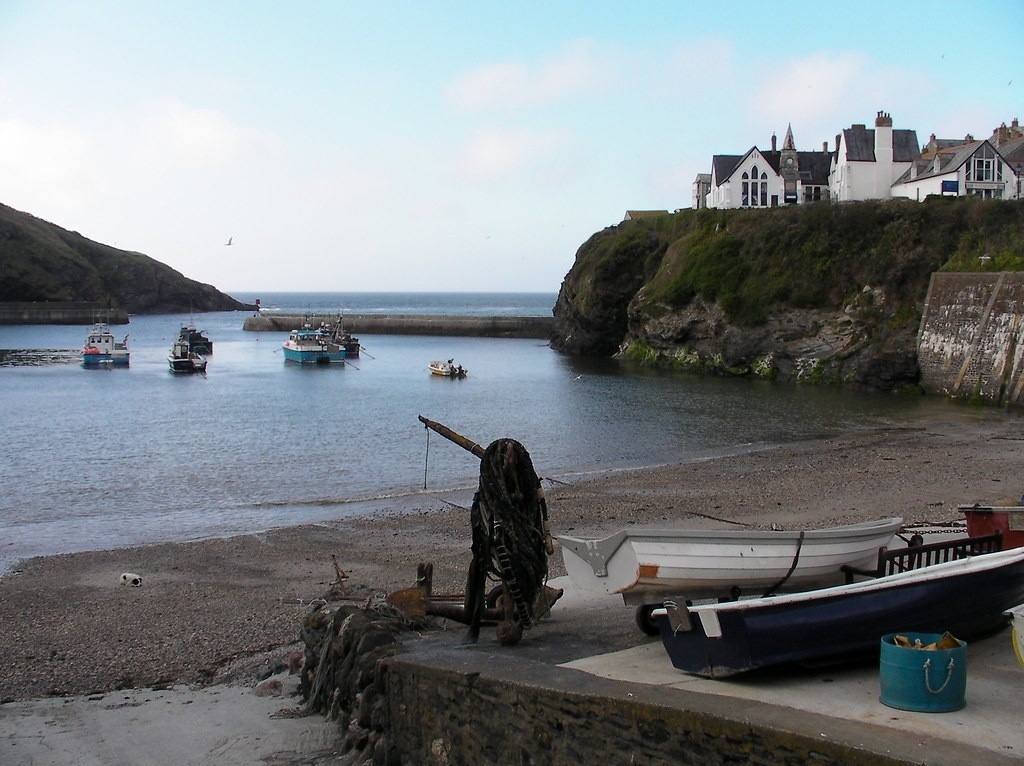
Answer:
[119,573,143,587]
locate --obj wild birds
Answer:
[224,237,233,246]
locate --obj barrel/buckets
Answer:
[879,633,968,713]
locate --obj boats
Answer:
[175,322,214,354]
[82,309,131,366]
[314,310,362,357]
[166,338,208,374]
[1000,604,1024,674]
[650,546,1024,681]
[281,301,347,364]
[428,359,467,378]
[554,517,905,608]
